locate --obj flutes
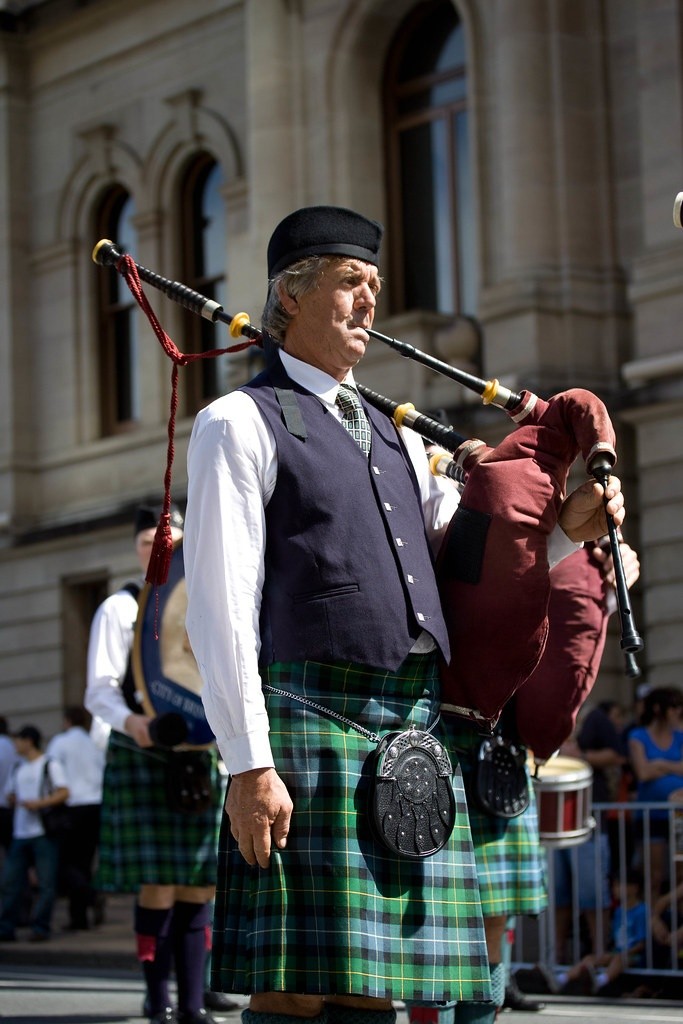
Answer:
[86,235,643,675]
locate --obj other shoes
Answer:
[143,997,179,1024]
[534,962,557,993]
[205,991,239,1012]
[29,932,46,942]
[176,1003,217,1024]
[501,985,543,1012]
[580,961,598,994]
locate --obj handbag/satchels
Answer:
[40,760,80,855]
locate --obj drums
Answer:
[527,753,595,847]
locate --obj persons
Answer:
[178,205,626,1024]
[0,500,683,1024]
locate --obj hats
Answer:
[267,205,384,280]
[10,724,42,744]
[134,503,184,536]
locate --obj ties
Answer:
[335,385,372,458]
